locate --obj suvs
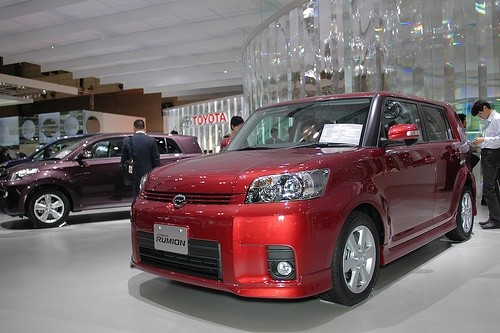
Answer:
[1,133,202,229]
[130,94,481,307]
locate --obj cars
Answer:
[0,135,102,173]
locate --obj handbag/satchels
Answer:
[120,160,135,176]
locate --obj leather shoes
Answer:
[482,223,498,229]
[479,222,487,225]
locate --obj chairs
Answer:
[301,119,334,142]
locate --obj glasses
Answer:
[478,111,483,116]
